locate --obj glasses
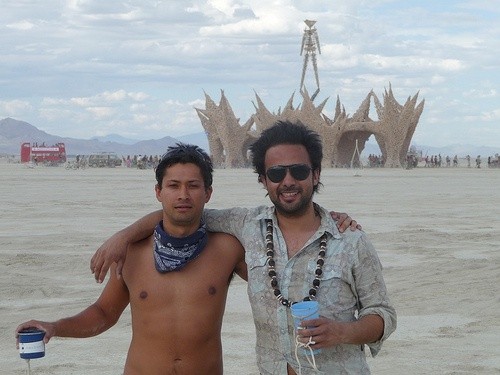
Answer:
[264,163,316,183]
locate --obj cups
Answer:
[16,330,45,360]
[290,300,320,356]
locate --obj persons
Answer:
[368,153,500,169]
[91,119,398,374]
[31,143,160,169]
[15,143,362,375]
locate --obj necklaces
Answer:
[265,219,328,308]
